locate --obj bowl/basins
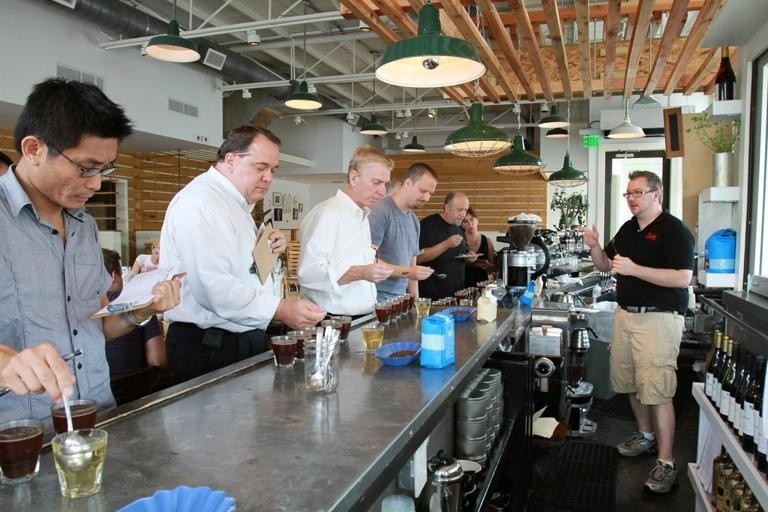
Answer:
[453,315,478,332]
[375,340,422,367]
[440,306,475,320]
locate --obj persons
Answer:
[416,191,477,303]
[293,145,396,335]
[367,163,439,300]
[582,171,695,498]
[461,207,498,287]
[1,153,15,177]
[158,124,328,384]
[1,78,182,445]
[89,238,187,397]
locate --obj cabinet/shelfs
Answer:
[694,98,742,291]
[686,380,765,511]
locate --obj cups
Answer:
[50,426,109,501]
[414,318,426,336]
[363,353,382,378]
[379,312,414,343]
[50,398,98,435]
[270,314,351,367]
[374,292,415,324]
[360,323,385,353]
[303,394,341,446]
[0,417,46,485]
[302,335,340,393]
[414,296,432,318]
[379,490,415,511]
[274,361,306,391]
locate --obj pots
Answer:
[374,362,420,384]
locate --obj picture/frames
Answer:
[272,190,304,222]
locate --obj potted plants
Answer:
[683,110,739,188]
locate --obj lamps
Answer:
[442,114,511,158]
[545,100,588,189]
[285,25,323,114]
[142,0,201,65]
[401,131,427,154]
[293,115,307,127]
[373,18,487,89]
[394,108,413,119]
[490,130,543,176]
[603,98,645,138]
[544,126,568,139]
[536,100,571,128]
[427,107,436,119]
[360,76,387,136]
[632,22,660,106]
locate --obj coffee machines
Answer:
[496,210,550,294]
[541,222,594,273]
[567,376,599,438]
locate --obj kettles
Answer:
[416,448,483,512]
[571,326,601,350]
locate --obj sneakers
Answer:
[642,458,679,494]
[616,431,657,457]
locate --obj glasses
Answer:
[36,135,116,178]
[622,189,655,198]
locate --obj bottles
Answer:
[712,439,764,512]
[433,277,508,305]
[711,45,739,102]
[703,326,767,477]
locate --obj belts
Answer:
[620,305,684,316]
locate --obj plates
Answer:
[111,485,237,511]
